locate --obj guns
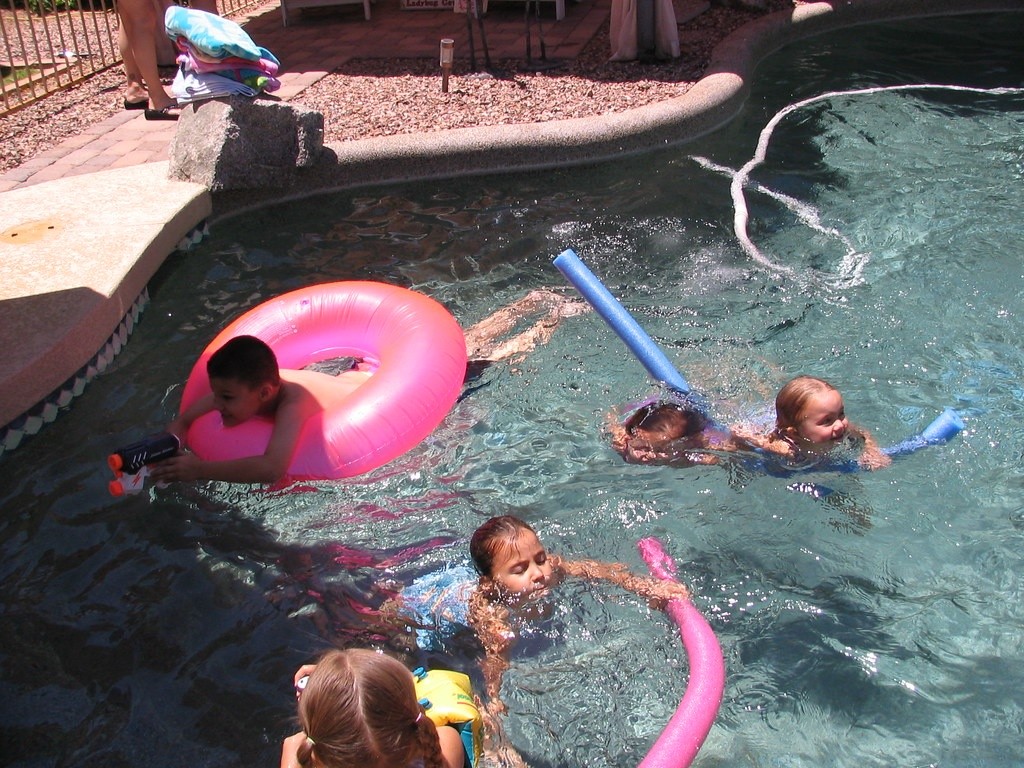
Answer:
[108,430,181,497]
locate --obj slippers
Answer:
[143,104,182,120]
[123,98,151,111]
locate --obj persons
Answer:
[280,647,466,767]
[382,515,690,737]
[600,398,764,467]
[731,376,891,471]
[108,0,185,120]
[147,291,593,487]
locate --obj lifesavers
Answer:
[552,248,965,478]
[637,537,725,768]
[180,280,467,482]
